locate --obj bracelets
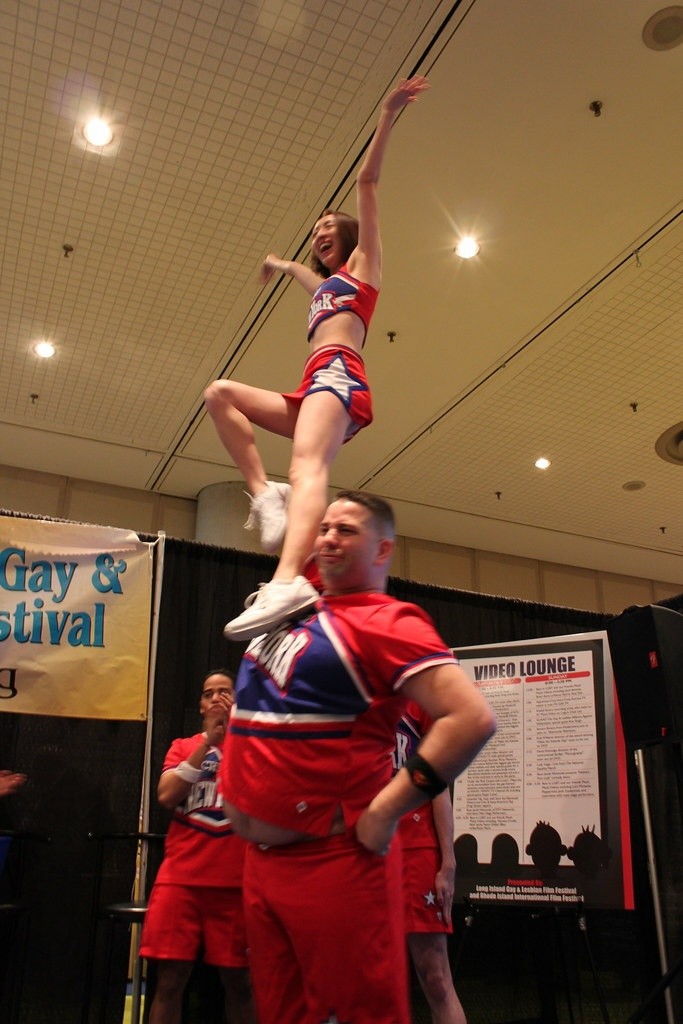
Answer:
[405,753,448,798]
[173,761,203,785]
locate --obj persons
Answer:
[204,76,431,641]
[217,492,497,1024]
[393,700,467,1024]
[137,670,249,1024]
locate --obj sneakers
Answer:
[223,575,320,638]
[243,479,292,552]
